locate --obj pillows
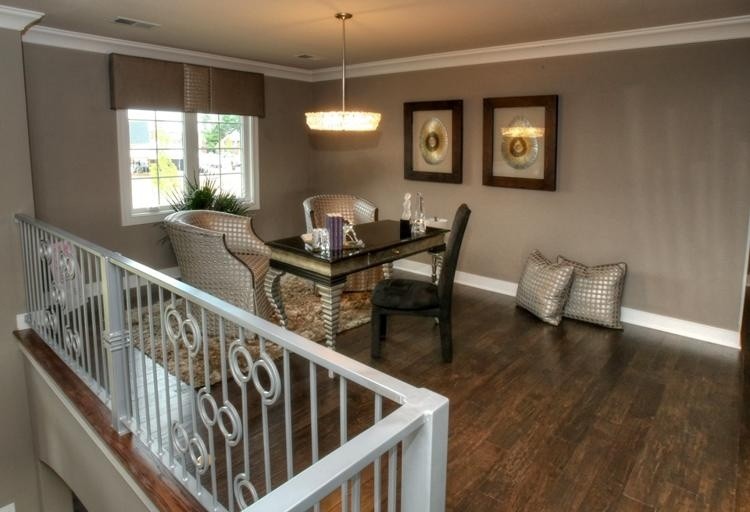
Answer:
[557,254,627,330]
[515,249,575,327]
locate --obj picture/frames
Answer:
[404,99,463,184]
[482,95,558,191]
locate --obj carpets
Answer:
[123,266,373,391]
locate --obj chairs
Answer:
[370,203,472,363]
[303,194,383,294]
[163,210,281,339]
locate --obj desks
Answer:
[265,219,451,378]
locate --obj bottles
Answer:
[414,192,426,235]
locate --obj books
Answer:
[325,212,344,250]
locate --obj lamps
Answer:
[304,11,381,132]
